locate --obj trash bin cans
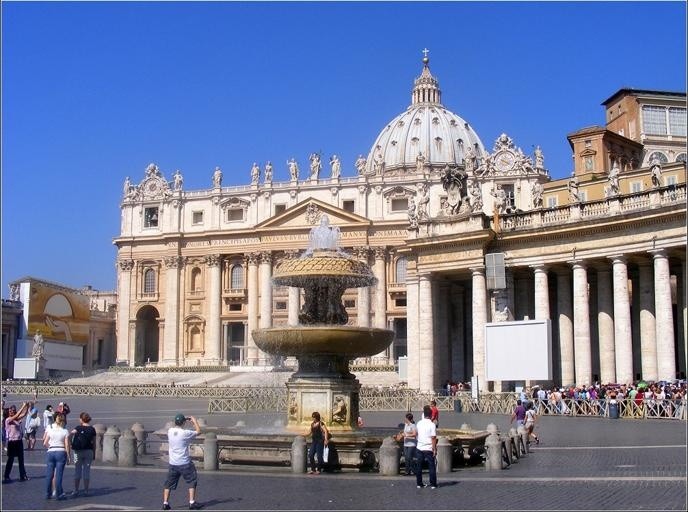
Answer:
[608,402,619,419]
[454,399,461,413]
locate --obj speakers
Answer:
[485,252,506,290]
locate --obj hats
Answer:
[174,412,187,422]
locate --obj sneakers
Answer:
[189,502,203,509]
[163,503,171,510]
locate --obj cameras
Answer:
[184,417,192,422]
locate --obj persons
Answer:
[416,152,425,171]
[212,166,223,188]
[531,181,543,208]
[32,328,44,357]
[287,157,299,180]
[355,154,367,175]
[308,153,322,179]
[0,383,97,501]
[607,162,620,193]
[374,154,385,174]
[124,170,184,202]
[264,161,273,182]
[485,145,544,176]
[330,155,342,178]
[465,147,476,167]
[45,316,73,341]
[357,411,363,427]
[162,412,205,510]
[251,162,260,184]
[394,379,688,489]
[300,411,330,476]
[332,397,345,422]
[650,153,664,188]
[567,172,579,202]
[407,182,507,226]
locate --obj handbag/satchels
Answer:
[323,433,332,440]
